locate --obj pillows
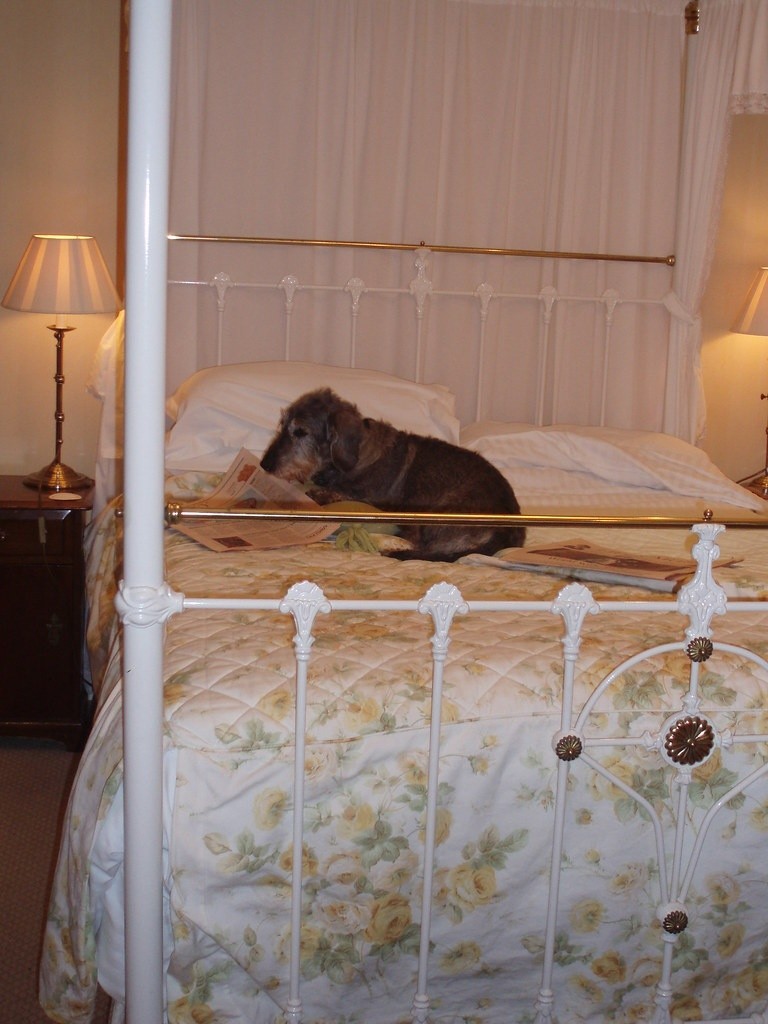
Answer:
[171,361,458,510]
[461,421,761,513]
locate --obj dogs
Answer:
[261,386,526,561]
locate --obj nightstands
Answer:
[0,475,93,744]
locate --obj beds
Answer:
[40,2,768,1024]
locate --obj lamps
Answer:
[0,236,120,493]
[699,266,768,496]
